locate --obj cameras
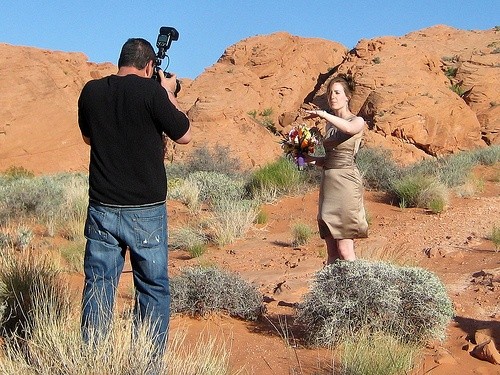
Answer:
[150,27,181,97]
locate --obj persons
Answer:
[77,39,192,375]
[296,73,368,266]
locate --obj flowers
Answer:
[276,123,321,173]
